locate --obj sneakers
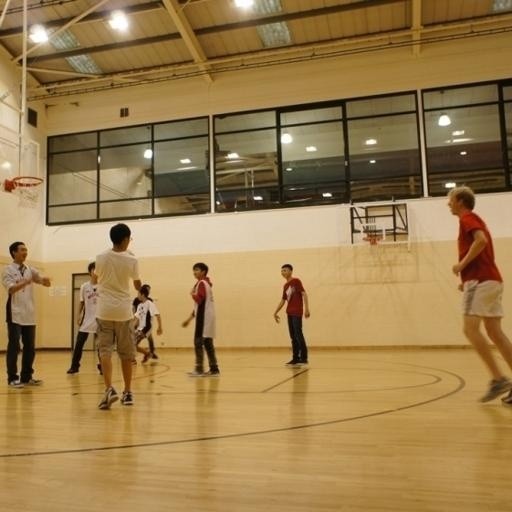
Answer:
[481,376,512,402]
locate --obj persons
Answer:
[447,183,512,403]
[133,284,159,359]
[181,263,219,377]
[1,240,51,388]
[94,223,144,410]
[66,262,103,375]
[274,264,313,365]
[132,287,163,363]
